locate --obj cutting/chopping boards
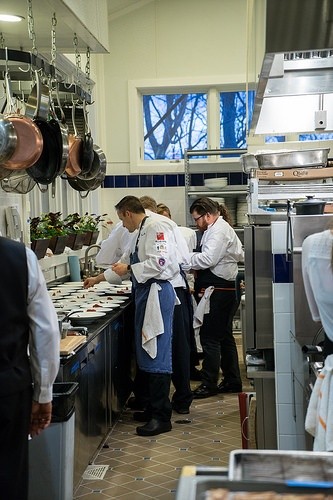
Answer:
[60,333,86,356]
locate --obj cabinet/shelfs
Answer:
[54,302,134,494]
[185,148,251,274]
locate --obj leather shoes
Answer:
[89,405,115,423]
[217,379,242,393]
[133,409,155,421]
[191,383,218,399]
[137,418,173,437]
[78,415,101,436]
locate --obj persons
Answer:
[302,217,333,453]
[83,196,244,436]
[0,236,60,500]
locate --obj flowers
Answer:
[28,211,113,237]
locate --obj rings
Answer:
[39,419,46,422]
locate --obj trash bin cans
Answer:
[29,382,78,500]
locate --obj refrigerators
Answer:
[239,213,277,451]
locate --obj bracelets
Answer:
[126,265,131,276]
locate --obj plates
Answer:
[204,177,229,191]
[48,281,133,322]
[189,194,250,228]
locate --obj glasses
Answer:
[193,211,206,221]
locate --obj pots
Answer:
[294,195,326,216]
[1,66,112,199]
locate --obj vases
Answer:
[31,228,100,260]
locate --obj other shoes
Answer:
[188,367,205,382]
[170,402,189,414]
